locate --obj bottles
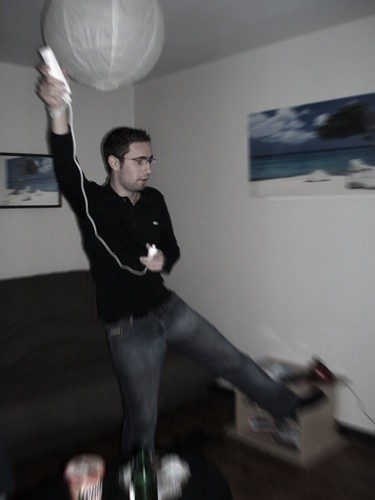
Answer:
[128,438,159,495]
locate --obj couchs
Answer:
[0,270,213,462]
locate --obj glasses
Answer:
[124,158,156,165]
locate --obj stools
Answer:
[216,355,352,471]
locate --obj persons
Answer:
[33,59,328,461]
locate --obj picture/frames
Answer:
[0,152,61,208]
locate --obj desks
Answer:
[4,436,234,499]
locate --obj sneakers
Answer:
[271,385,328,430]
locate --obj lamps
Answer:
[44,1,164,91]
